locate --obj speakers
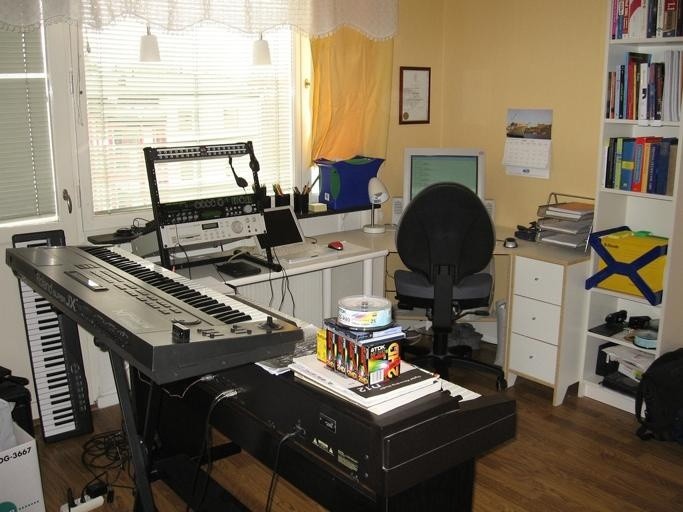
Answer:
[485,199,496,224]
[392,197,403,225]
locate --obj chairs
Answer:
[394,181,508,393]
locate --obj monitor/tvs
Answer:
[403,146,486,218]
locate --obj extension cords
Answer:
[60,487,105,512]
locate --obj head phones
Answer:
[228,142,260,186]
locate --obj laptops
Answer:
[253,205,338,264]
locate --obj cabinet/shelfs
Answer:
[578,0,683,417]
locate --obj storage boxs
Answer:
[0,421,47,511]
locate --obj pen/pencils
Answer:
[273,184,284,196]
[293,176,320,195]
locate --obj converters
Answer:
[86,481,108,498]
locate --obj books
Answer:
[538,219,593,234]
[321,324,407,348]
[547,201,594,216]
[541,232,588,247]
[321,315,402,341]
[544,209,594,220]
[604,0,682,196]
[287,350,442,416]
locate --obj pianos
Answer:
[4,237,304,512]
[11,228,93,440]
[129,310,519,512]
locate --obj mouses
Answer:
[328,241,343,250]
[504,237,518,248]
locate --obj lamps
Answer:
[253,31,270,65]
[364,177,390,234]
[139,18,161,63]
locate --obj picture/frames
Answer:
[399,67,430,125]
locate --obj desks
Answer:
[144,238,390,330]
[313,224,590,406]
